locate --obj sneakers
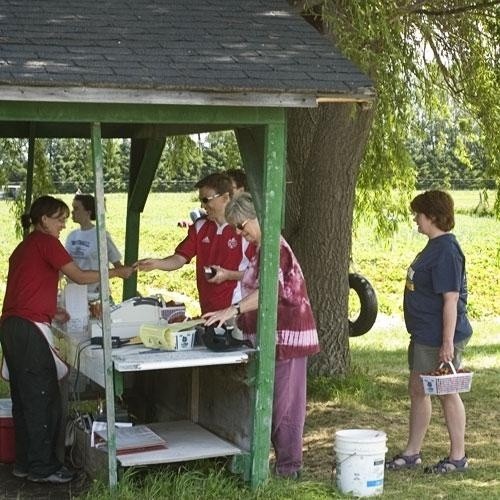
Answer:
[12,468,30,477]
[26,470,73,483]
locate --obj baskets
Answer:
[139,310,196,352]
[155,294,186,322]
[419,361,474,396]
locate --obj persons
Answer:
[1,194,135,483]
[384,189,474,476]
[223,168,251,202]
[64,191,126,308]
[201,192,320,483]
[130,173,251,341]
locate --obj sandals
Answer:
[424,456,469,474]
[385,454,424,469]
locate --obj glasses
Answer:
[233,219,249,230]
[199,193,221,204]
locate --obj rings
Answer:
[214,316,218,318]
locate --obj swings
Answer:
[348,233,377,337]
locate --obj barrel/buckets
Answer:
[0,398,18,465]
[334,430,388,497]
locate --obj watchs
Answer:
[232,303,241,317]
